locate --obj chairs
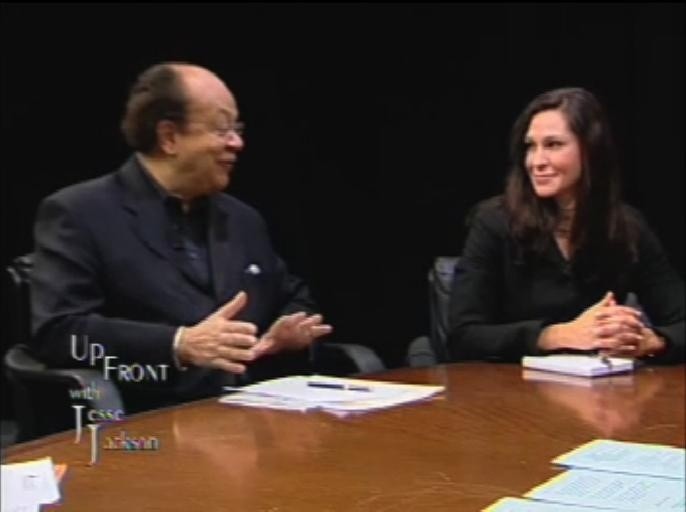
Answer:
[2,248,387,441]
[403,253,459,368]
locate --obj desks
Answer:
[0,359,685,512]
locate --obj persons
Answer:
[26,57,337,411]
[443,86,686,369]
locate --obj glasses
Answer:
[183,118,244,139]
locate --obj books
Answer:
[519,348,638,379]
[216,371,451,417]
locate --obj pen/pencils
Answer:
[307,381,369,393]
[600,355,614,370]
